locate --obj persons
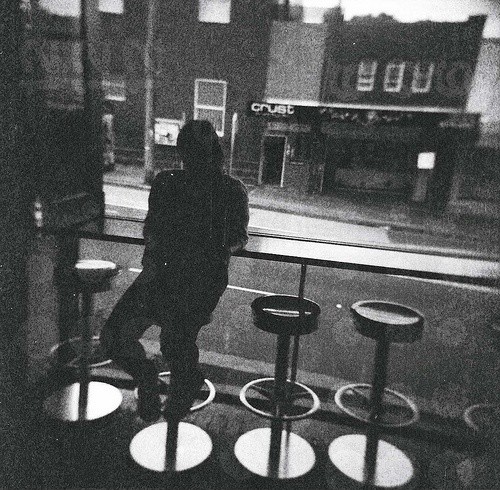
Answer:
[104,118,249,427]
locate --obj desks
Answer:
[70,216,499,438]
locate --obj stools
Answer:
[332,299,425,489]
[233,295,325,487]
[131,304,219,477]
[38,257,123,425]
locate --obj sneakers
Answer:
[135,361,160,425]
[164,367,206,421]
